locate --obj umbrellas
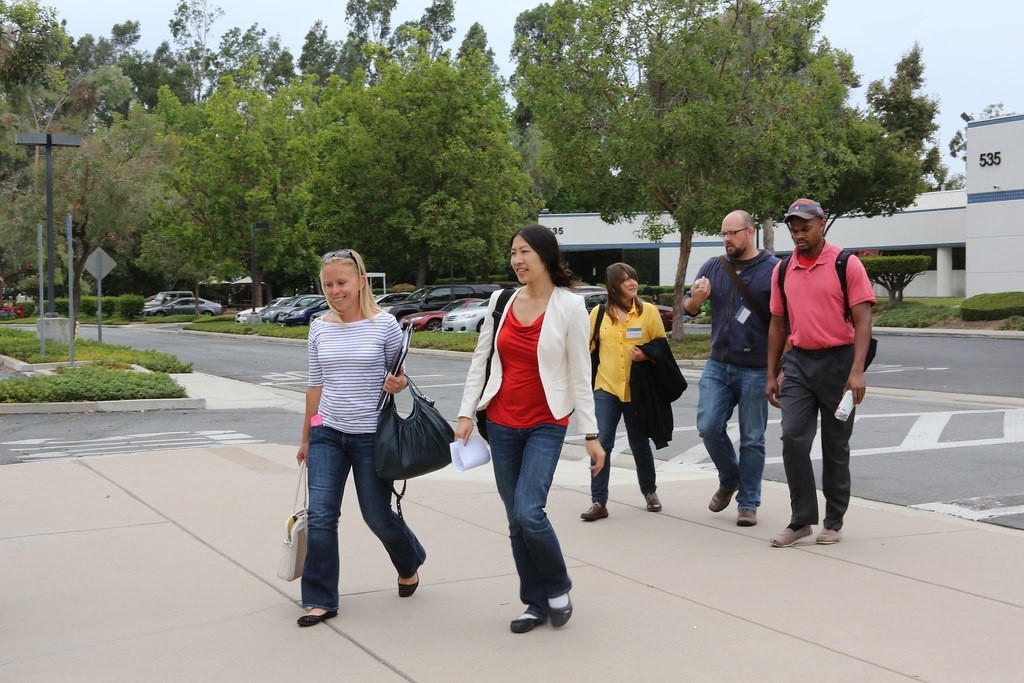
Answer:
[232,275,266,300]
[198,276,231,296]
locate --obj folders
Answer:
[377,326,413,411]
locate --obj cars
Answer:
[382,284,503,322]
[375,292,411,306]
[284,297,329,327]
[442,296,491,333]
[142,297,224,316]
[235,298,291,324]
[400,298,488,332]
[578,292,675,332]
[260,295,325,326]
[309,310,333,327]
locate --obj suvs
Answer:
[144,291,194,309]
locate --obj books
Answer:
[376,327,412,411]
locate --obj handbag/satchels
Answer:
[590,339,601,390]
[277,458,308,582]
[375,374,454,525]
[475,409,489,443]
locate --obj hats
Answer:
[784,197,826,221]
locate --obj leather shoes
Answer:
[645,493,662,511]
[709,484,740,512]
[580,503,608,520]
[737,508,757,527]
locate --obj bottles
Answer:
[834,390,855,422]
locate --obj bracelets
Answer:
[585,434,598,440]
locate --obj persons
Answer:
[682,210,794,527]
[643,281,654,296]
[221,296,229,312]
[766,199,877,548]
[580,263,668,521]
[297,249,426,626]
[454,225,605,634]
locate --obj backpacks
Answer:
[779,249,878,373]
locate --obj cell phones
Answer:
[311,413,322,427]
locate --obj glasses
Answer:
[321,249,359,269]
[718,227,749,237]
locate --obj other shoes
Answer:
[816,528,842,544]
[770,523,813,548]
[397,571,419,597]
[510,614,542,633]
[547,591,572,628]
[297,610,338,626]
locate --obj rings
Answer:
[698,286,704,288]
